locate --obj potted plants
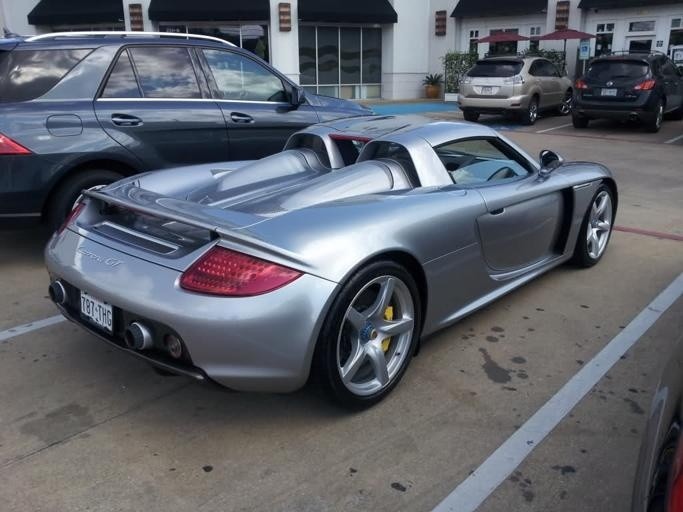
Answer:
[421,74,444,98]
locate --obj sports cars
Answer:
[44,115,618,411]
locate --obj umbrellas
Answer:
[471,28,529,44]
[530,28,598,59]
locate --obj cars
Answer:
[573,54,682,132]
[0,31,373,236]
[632,335,682,512]
[458,54,574,125]
